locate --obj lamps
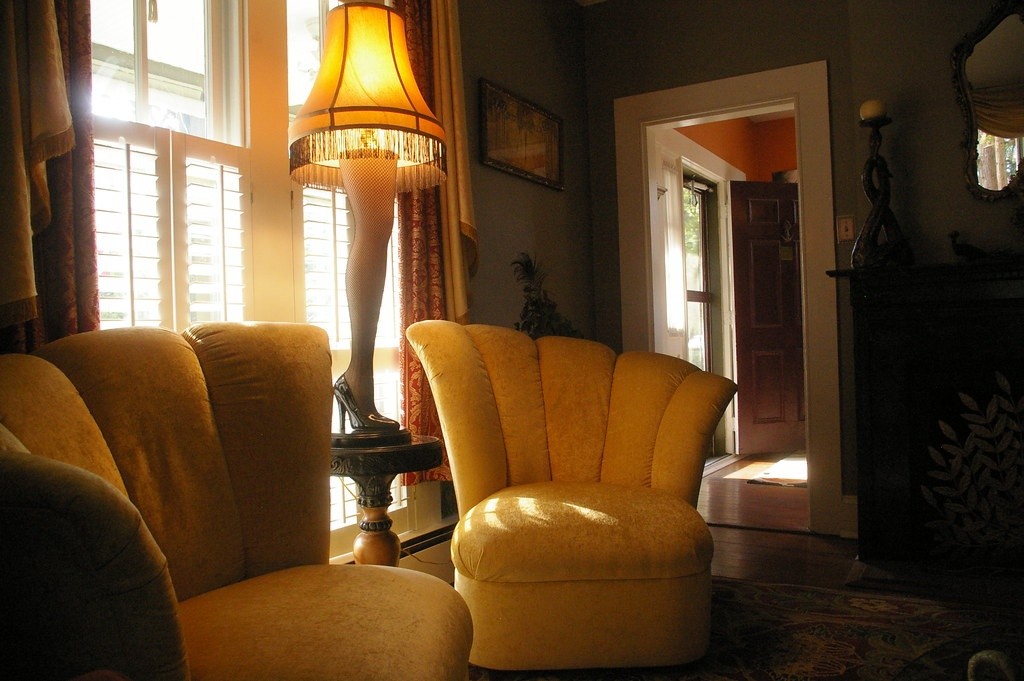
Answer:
[286,3,450,444]
[851,99,916,267]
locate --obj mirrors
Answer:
[952,0,1024,224]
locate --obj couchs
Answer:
[0,322,473,681]
[404,320,739,670]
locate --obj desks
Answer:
[329,436,441,566]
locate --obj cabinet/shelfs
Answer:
[826,257,1024,566]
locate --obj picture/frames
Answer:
[478,77,564,190]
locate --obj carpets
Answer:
[468,573,1024,681]
[747,450,808,487]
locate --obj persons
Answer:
[333,148,399,434]
